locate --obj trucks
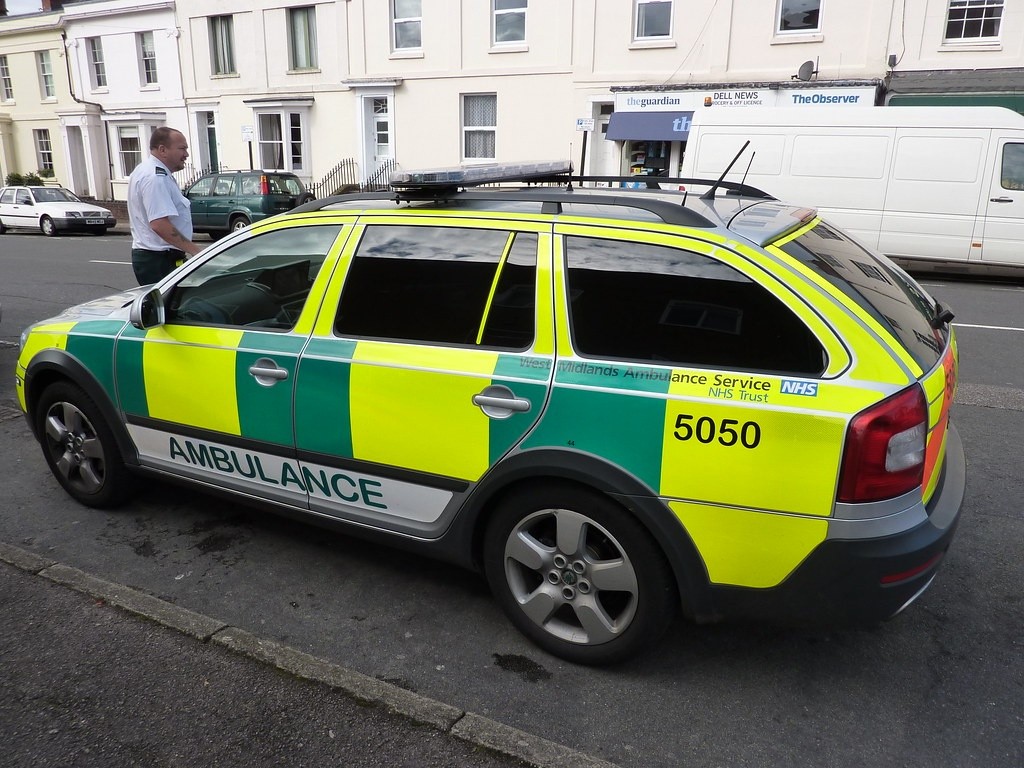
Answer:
[680,107,1024,282]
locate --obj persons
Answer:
[127,127,204,287]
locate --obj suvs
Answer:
[15,159,969,669]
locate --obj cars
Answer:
[182,169,317,240]
[1,185,118,238]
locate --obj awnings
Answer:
[604,111,695,141]
